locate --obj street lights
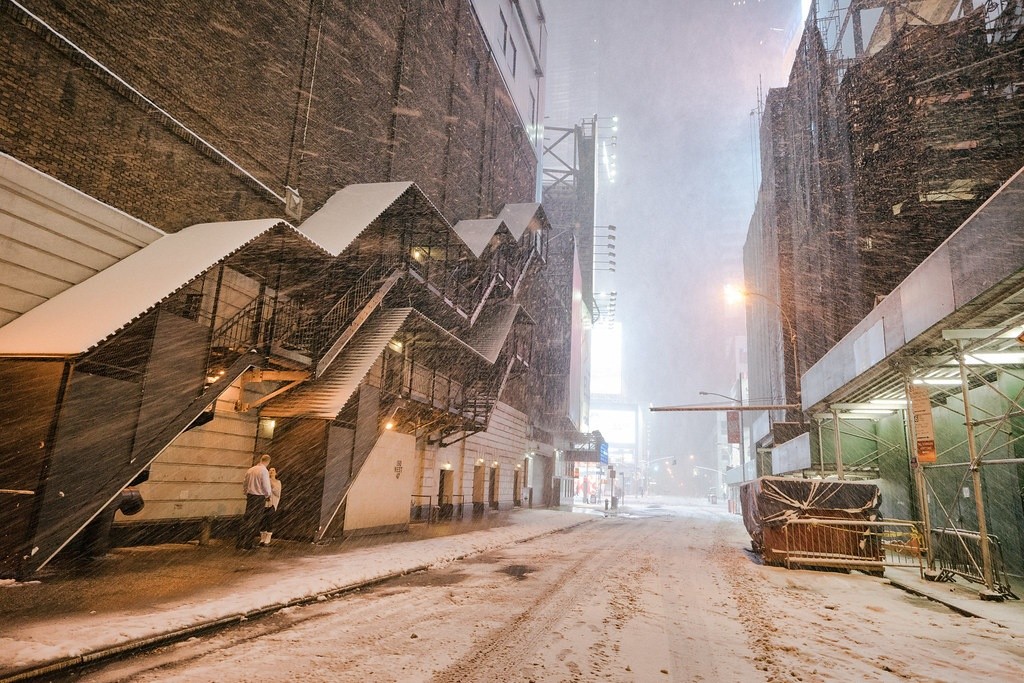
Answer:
[698,391,746,484]
[723,286,802,417]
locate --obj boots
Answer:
[256,531,273,546]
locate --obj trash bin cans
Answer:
[710,495,717,504]
[728,500,737,512]
[612,496,618,509]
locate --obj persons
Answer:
[236,454,271,551]
[259,467,281,546]
